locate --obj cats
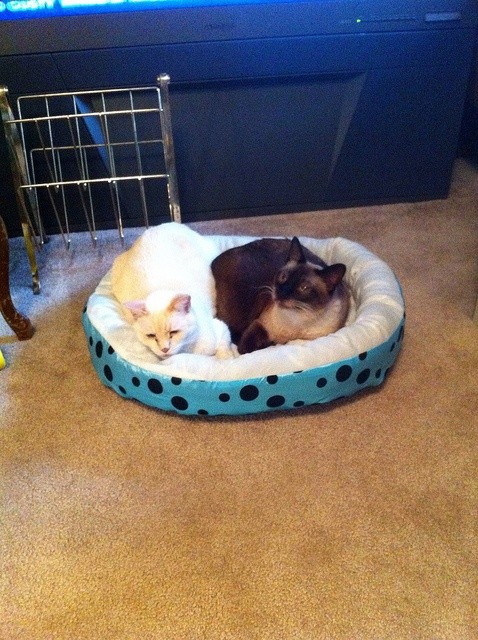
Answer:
[208,235,357,355]
[109,222,235,360]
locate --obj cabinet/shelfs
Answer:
[0,28,476,236]
